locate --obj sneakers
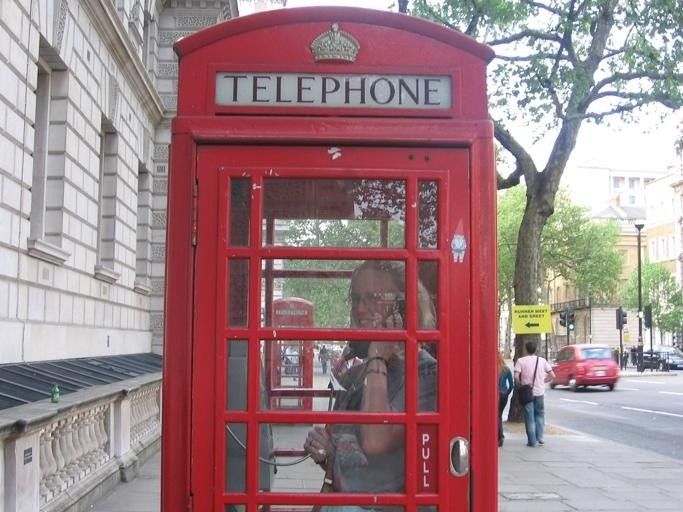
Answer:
[526,437,544,446]
[498,437,504,447]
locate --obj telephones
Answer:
[349,300,406,359]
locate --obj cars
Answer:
[549,343,621,392]
[641,345,683,371]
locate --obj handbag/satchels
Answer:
[518,384,533,405]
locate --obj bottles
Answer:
[51,384,59,403]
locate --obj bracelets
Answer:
[363,369,388,377]
[366,357,388,368]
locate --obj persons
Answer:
[630,344,638,366]
[497,351,513,447]
[318,345,329,374]
[304,260,438,512]
[515,341,556,444]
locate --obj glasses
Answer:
[344,291,397,309]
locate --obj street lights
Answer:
[634,219,646,372]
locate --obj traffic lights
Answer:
[615,307,628,330]
[643,304,651,328]
[567,312,575,331]
[560,312,566,327]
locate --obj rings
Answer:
[310,439,315,446]
